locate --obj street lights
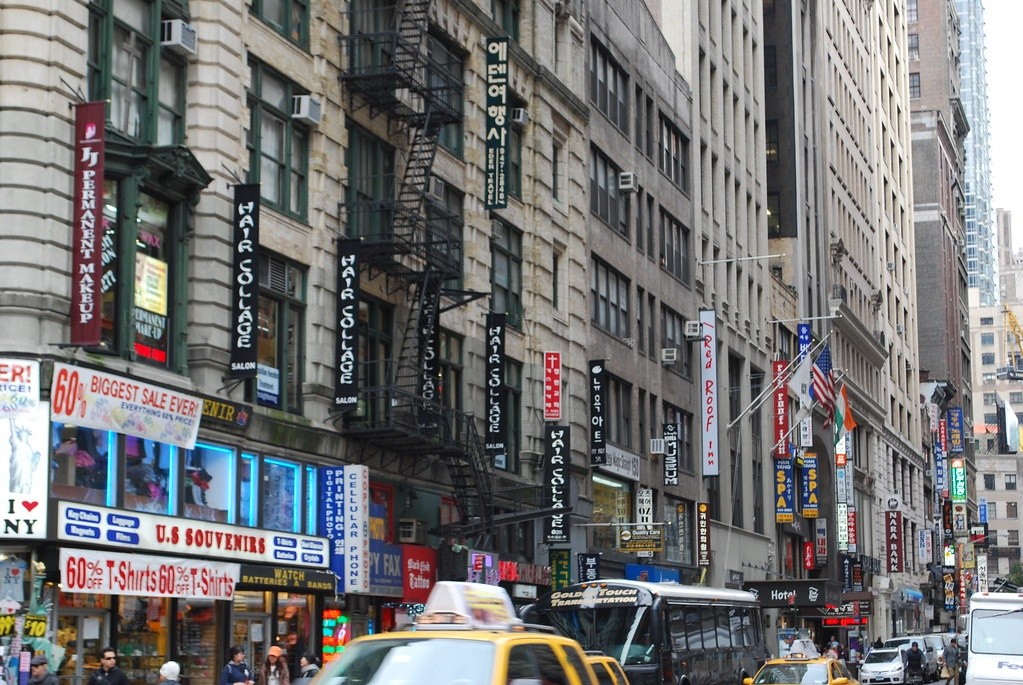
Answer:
[962,533,1009,614]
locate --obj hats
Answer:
[268,646,281,657]
[159,661,181,681]
[231,646,244,655]
[31,654,47,665]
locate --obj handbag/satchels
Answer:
[940,664,950,678]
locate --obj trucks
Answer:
[958,591,1023,684]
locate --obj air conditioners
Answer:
[961,330,964,336]
[619,172,639,192]
[894,488,899,494]
[511,107,529,126]
[399,517,430,546]
[965,318,969,324]
[426,175,446,199]
[684,321,703,337]
[291,96,320,127]
[896,324,903,333]
[962,389,976,444]
[906,363,912,371]
[926,469,933,476]
[158,18,198,56]
[888,262,895,271]
[904,287,909,294]
[649,438,670,457]
[660,348,680,362]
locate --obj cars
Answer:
[927,633,960,669]
[584,651,631,685]
[742,638,860,685]
[305,580,599,685]
[859,647,908,685]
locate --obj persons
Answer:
[159,661,181,685]
[86,647,128,685]
[902,641,926,685]
[942,630,968,685]
[300,648,320,678]
[777,634,884,666]
[257,646,290,685]
[220,645,255,685]
[26,655,59,685]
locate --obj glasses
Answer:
[31,663,45,667]
[104,657,116,660]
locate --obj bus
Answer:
[516,578,764,685]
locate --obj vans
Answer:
[884,635,940,684]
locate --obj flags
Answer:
[789,342,857,447]
